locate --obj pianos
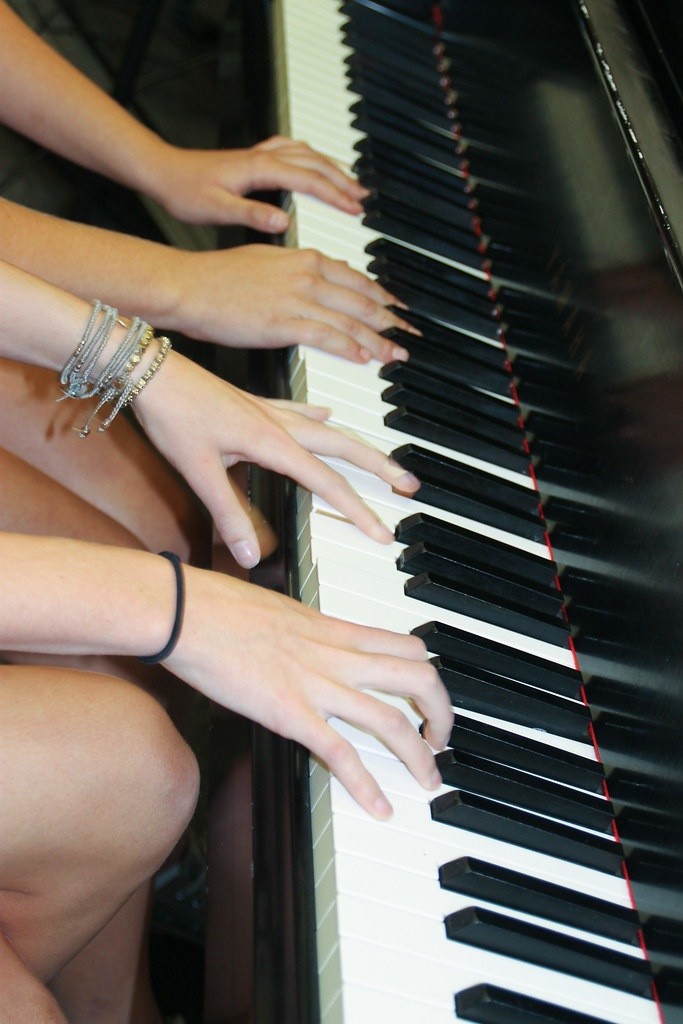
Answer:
[183,2,680,1015]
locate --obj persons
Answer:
[0,0,452,1024]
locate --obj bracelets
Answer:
[55,298,171,439]
[136,552,186,665]
